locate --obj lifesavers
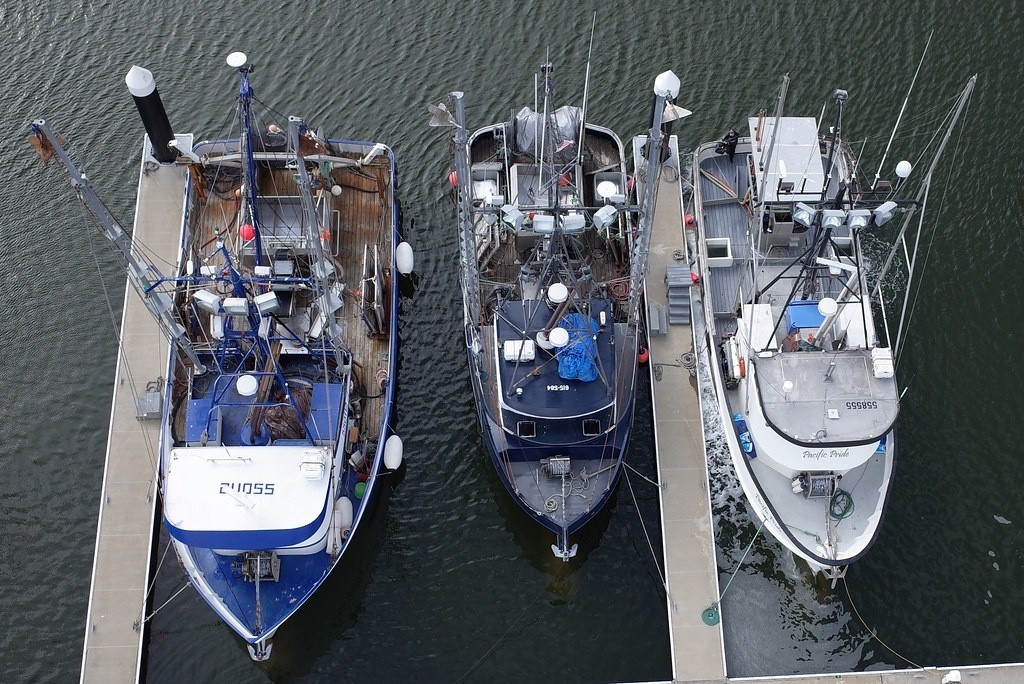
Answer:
[740,357,745,377]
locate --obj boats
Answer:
[425,9,681,565]
[691,26,980,568]
[25,47,411,662]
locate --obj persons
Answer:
[760,206,773,235]
[722,130,741,164]
